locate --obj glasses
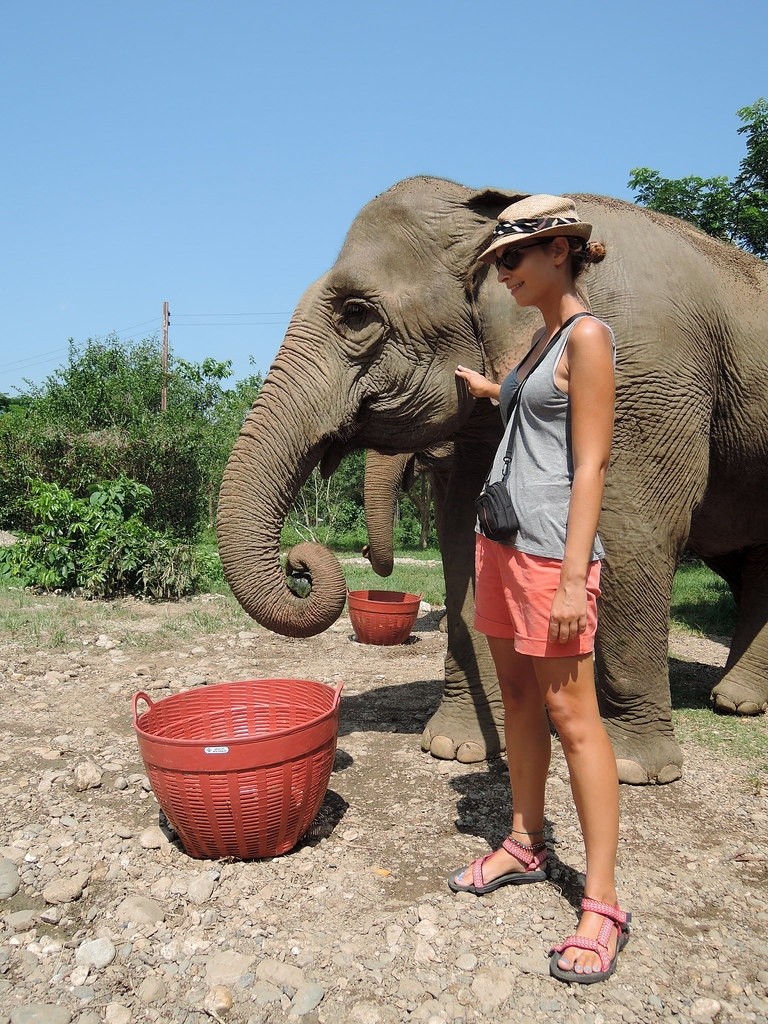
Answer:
[494,245,541,273]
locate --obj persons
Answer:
[448,193,631,980]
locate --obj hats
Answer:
[477,194,592,264]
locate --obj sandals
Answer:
[448,837,550,894]
[549,896,631,984]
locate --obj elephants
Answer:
[214,176,767,786]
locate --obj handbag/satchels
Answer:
[475,482,520,543]
